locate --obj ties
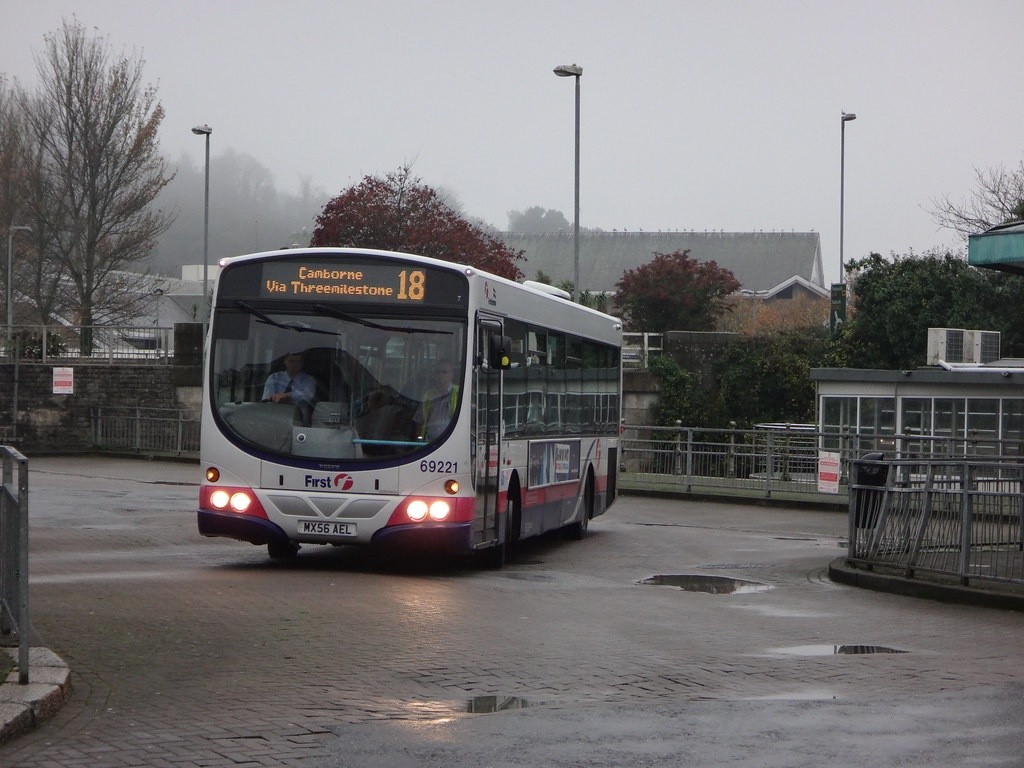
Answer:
[286,378,294,394]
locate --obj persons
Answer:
[412,359,460,442]
[261,350,318,426]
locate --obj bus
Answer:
[196,244,622,570]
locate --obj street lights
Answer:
[192,122,213,341]
[836,108,856,320]
[7,223,34,342]
[553,63,585,303]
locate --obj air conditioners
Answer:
[927,328,966,365]
[967,330,1001,364]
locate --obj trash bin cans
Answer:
[855,453,889,530]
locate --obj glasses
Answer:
[434,368,452,375]
